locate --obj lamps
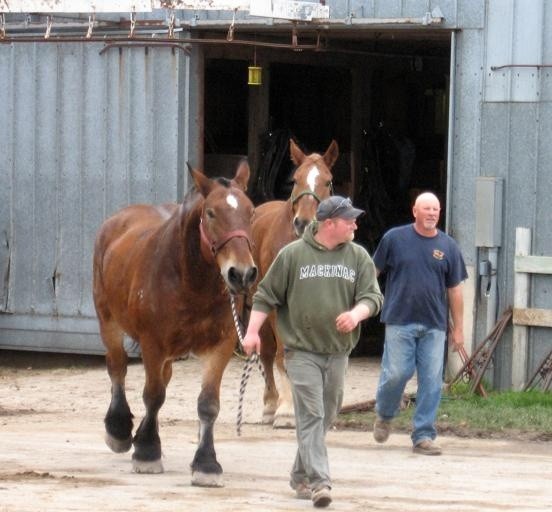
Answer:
[248,33,262,85]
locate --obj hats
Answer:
[316,195,368,222]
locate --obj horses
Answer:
[89,156,260,488]
[254,137,343,429]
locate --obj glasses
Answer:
[326,196,353,220]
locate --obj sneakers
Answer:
[310,483,334,507]
[411,435,445,456]
[288,475,313,499]
[370,415,396,442]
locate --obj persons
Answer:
[241,195,387,508]
[369,191,469,455]
